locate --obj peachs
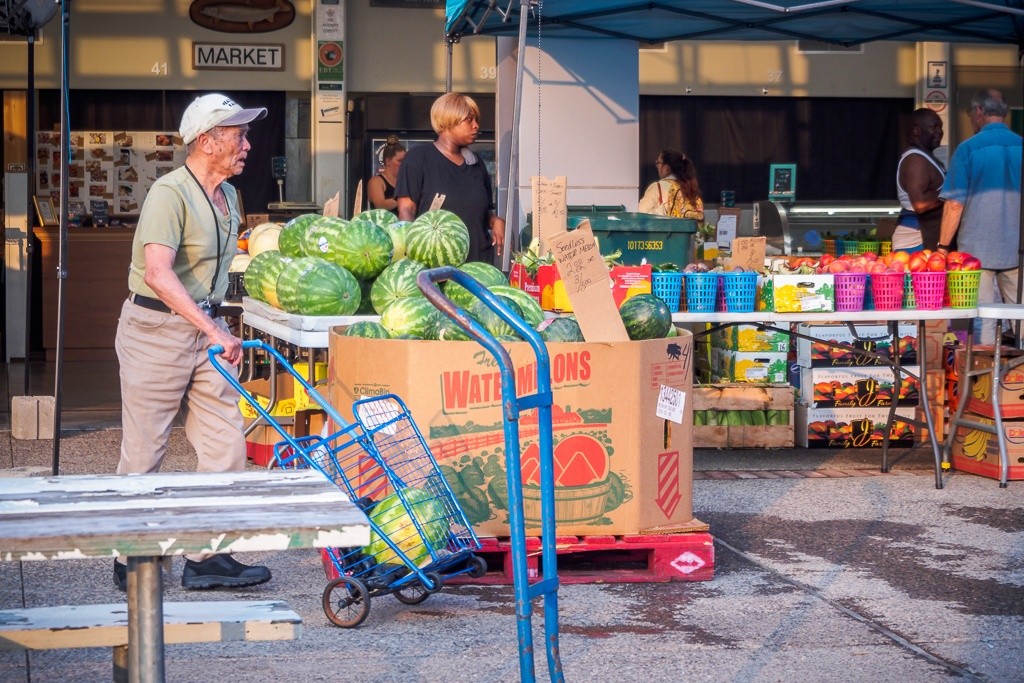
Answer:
[795,249,981,275]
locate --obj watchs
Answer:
[936,242,950,249]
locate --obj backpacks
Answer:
[661,178,705,227]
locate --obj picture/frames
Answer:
[33,195,59,227]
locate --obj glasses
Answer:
[655,160,663,167]
[967,107,974,116]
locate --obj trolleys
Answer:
[206,338,488,630]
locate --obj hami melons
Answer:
[228,222,282,271]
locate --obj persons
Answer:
[934,84,1023,347]
[393,90,505,266]
[112,93,271,594]
[892,107,947,254]
[368,134,408,216]
[640,148,705,263]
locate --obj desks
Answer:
[976,300,1024,488]
[665,302,978,492]
[0,468,370,683]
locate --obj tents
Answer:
[443,0,1023,276]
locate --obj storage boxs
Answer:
[246,211,302,232]
[324,323,698,538]
[757,269,836,316]
[690,319,1024,490]
[236,361,328,471]
[509,259,655,317]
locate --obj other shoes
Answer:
[113,557,127,591]
[181,555,272,589]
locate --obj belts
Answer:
[127,292,244,319]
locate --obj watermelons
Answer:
[359,488,450,570]
[618,293,677,340]
[242,207,583,342]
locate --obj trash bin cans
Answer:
[518,203,699,273]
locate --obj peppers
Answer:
[651,262,680,273]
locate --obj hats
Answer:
[179,94,268,145]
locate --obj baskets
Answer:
[652,272,758,313]
[833,270,982,312]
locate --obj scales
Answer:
[265,156,322,212]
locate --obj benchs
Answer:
[0,599,303,683]
